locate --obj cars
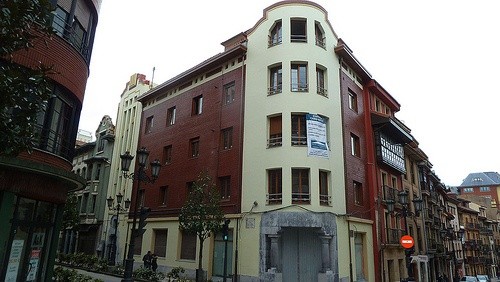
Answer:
[458,274,491,282]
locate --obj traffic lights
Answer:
[137,205,152,237]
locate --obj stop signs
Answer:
[400,235,415,249]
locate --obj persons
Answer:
[142,251,158,272]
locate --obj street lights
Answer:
[106,192,132,266]
[119,145,163,282]
[439,223,465,282]
[383,189,424,281]
